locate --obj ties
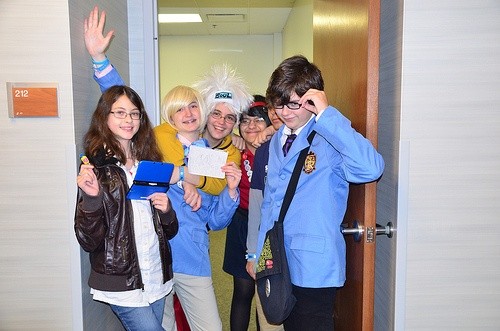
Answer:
[282,133,297,156]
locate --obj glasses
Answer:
[263,106,274,112]
[110,110,143,120]
[211,112,236,124]
[272,101,302,110]
[240,117,266,126]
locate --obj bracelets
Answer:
[179,166,185,181]
[91,57,109,71]
[231,190,237,200]
[246,254,256,261]
[177,181,183,189]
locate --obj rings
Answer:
[78,173,81,176]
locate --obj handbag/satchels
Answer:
[255,221,297,326]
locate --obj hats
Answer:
[251,94,267,107]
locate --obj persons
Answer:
[148,63,254,331]
[254,55,385,331]
[74,85,178,331]
[222,94,276,331]
[83,5,242,331]
[245,88,285,331]
